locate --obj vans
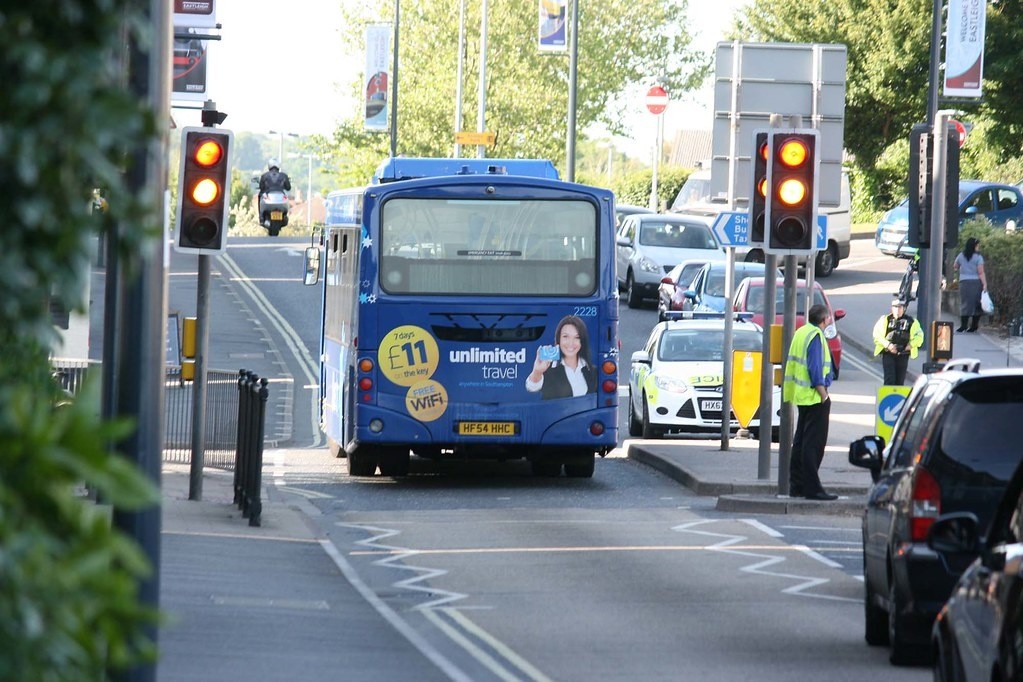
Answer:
[673,166,852,280]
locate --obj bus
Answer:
[303,156,623,482]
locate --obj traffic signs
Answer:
[712,212,830,255]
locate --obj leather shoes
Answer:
[806,489,838,500]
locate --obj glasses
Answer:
[969,237,977,243]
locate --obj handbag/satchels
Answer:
[980,290,994,312]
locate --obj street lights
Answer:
[266,128,300,173]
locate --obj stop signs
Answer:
[946,120,973,154]
[646,85,670,114]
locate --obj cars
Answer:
[873,178,1023,259]
[657,259,703,322]
[627,311,798,442]
[734,277,849,379]
[923,459,1023,682]
[614,204,654,238]
[615,215,726,309]
[685,263,786,324]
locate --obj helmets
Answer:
[268,157,281,170]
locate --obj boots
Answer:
[968,315,980,333]
[955,316,968,333]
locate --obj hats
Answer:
[889,292,907,306]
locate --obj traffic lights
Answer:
[748,129,774,248]
[173,125,229,253]
[930,320,959,364]
[764,128,821,254]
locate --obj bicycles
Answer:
[895,263,919,314]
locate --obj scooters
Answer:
[252,178,289,237]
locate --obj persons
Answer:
[873,301,924,386]
[527,315,593,400]
[258,158,291,227]
[783,304,839,499]
[955,238,987,332]
[642,228,662,244]
[680,229,707,247]
[996,543,1023,577]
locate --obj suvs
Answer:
[846,359,1023,669]
